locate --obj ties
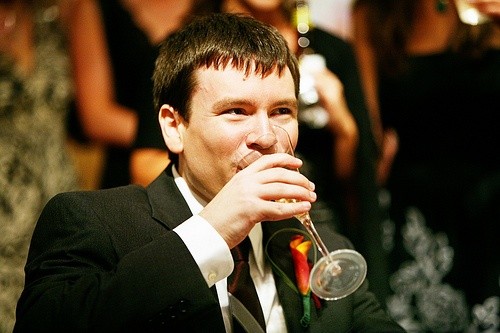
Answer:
[227,243,266,332]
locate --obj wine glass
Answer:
[234,125,367,299]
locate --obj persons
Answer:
[13,12,407,333]
[0,0,75,333]
[244,0,372,274]
[71,0,248,189]
[355,0,481,313]
